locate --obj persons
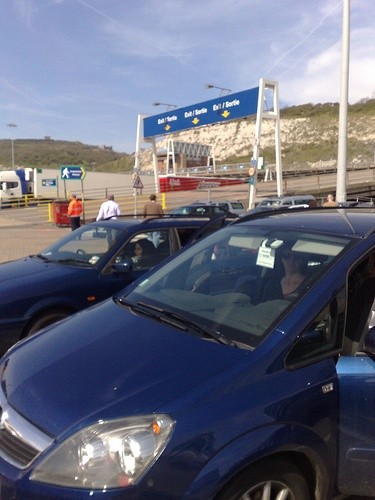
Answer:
[263,241,308,305]
[95,193,121,221]
[324,195,337,206]
[132,239,157,262]
[66,194,84,231]
[144,194,164,220]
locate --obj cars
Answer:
[163,199,245,221]
[0,204,375,500]
[0,212,243,358]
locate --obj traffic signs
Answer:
[60,165,86,180]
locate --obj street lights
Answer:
[7,123,18,171]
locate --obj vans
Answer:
[255,194,318,210]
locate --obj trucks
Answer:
[0,165,70,209]
